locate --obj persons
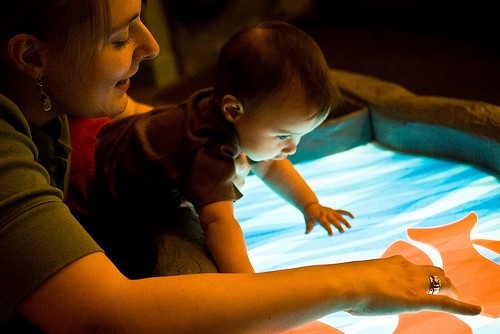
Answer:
[0,0,483,333]
[67,20,356,274]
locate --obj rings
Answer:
[428,273,443,295]
